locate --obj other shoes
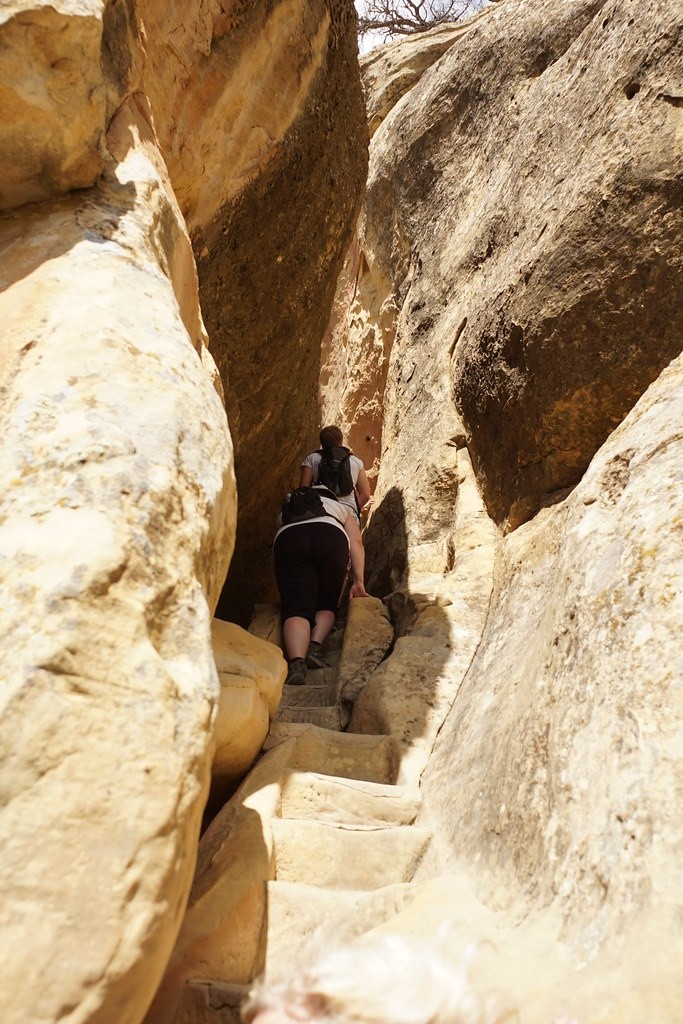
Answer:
[306,642,332,670]
[285,657,308,686]
[331,621,338,632]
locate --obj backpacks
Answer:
[313,444,361,520]
[276,486,347,529]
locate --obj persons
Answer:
[272,482,368,686]
[298,424,370,525]
